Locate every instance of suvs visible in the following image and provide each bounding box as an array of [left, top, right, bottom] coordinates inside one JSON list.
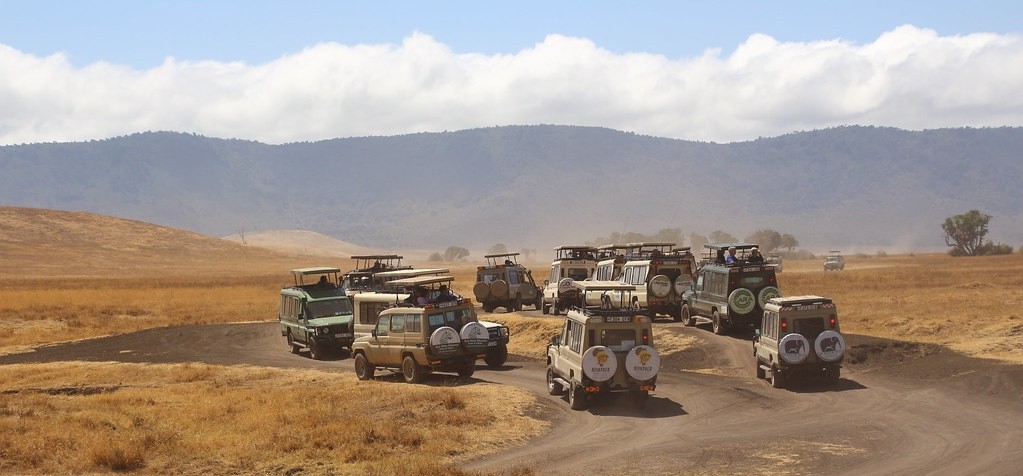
[[472, 252, 545, 313], [823, 250, 846, 271], [753, 295, 846, 387], [279, 267, 353, 359], [334, 252, 510, 370], [351, 275, 490, 384], [764, 249, 784, 274], [540, 240, 701, 321], [547, 281, 660, 411], [681, 242, 783, 334]]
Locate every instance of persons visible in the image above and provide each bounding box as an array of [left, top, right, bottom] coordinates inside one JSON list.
[[415, 287, 427, 304], [748, 247, 763, 264], [437, 285, 450, 300], [317, 276, 337, 296], [727, 247, 738, 263]]
